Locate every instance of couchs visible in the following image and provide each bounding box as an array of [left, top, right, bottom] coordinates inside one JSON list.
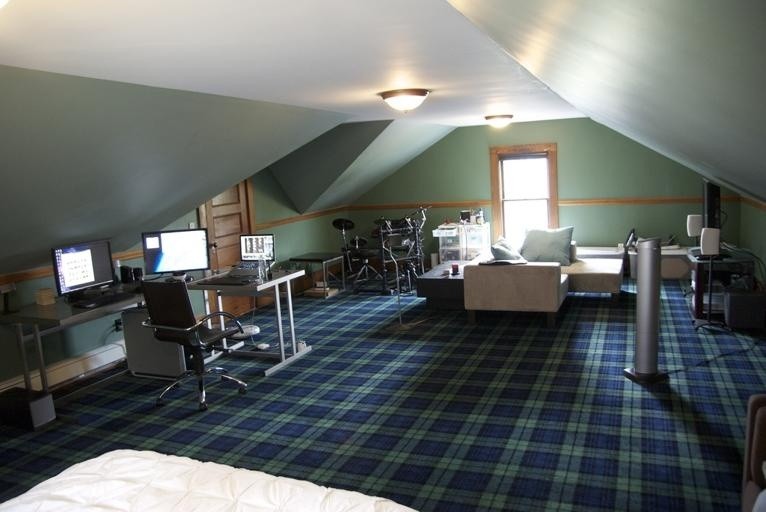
[[463, 241, 624, 322]]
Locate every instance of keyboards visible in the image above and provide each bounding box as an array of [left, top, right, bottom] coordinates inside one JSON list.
[[73, 293, 135, 309]]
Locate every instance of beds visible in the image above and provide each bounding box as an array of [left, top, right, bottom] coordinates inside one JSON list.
[[0, 449, 419, 512]]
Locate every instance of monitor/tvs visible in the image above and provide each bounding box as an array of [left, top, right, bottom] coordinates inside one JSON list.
[[692, 176, 732, 260], [142, 228, 210, 282], [50, 237, 115, 303]]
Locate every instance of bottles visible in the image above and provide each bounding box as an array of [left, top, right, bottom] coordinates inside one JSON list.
[[113, 259, 121, 281], [258, 254, 267, 280]]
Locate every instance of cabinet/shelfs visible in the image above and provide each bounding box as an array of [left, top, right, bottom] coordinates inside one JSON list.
[[465, 222, 490, 261], [432, 222, 465, 263]]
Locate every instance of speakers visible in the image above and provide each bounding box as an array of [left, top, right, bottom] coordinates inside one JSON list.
[[687, 214, 702, 237], [700, 228, 720, 255], [0, 387, 56, 431], [723, 290, 766, 329], [120, 265, 133, 283], [133, 267, 142, 281]]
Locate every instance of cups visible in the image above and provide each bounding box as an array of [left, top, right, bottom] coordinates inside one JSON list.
[[442, 269, 449, 275], [452, 264, 458, 273], [476, 215, 482, 225]]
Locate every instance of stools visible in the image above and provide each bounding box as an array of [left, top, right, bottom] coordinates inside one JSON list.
[[353, 249, 384, 285]]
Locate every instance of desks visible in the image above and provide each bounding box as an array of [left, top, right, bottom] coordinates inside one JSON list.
[[289, 252, 345, 300], [0, 269, 229, 392], [687, 246, 755, 319], [187, 269, 312, 376]]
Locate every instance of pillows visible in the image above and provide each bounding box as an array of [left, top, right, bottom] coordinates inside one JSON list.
[[491, 237, 525, 261], [521, 225, 574, 266]]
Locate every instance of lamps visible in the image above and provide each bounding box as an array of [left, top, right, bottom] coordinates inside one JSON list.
[[486, 115, 513, 129], [380, 89, 429, 110], [692, 228, 725, 331], [683, 214, 703, 297], [388, 234, 413, 331]]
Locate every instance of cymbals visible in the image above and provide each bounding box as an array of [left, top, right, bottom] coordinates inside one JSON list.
[[374, 219, 400, 226], [332, 219, 354, 230], [350, 238, 368, 246]]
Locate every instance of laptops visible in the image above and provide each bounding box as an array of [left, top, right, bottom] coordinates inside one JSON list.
[[228, 234, 275, 276]]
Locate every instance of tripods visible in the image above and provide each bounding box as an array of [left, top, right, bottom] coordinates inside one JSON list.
[[695, 253, 725, 331]]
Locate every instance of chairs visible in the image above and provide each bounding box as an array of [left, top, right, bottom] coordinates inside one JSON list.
[[141, 281, 248, 410]]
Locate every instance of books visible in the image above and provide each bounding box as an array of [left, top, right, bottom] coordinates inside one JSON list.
[[660, 241, 680, 251]]
